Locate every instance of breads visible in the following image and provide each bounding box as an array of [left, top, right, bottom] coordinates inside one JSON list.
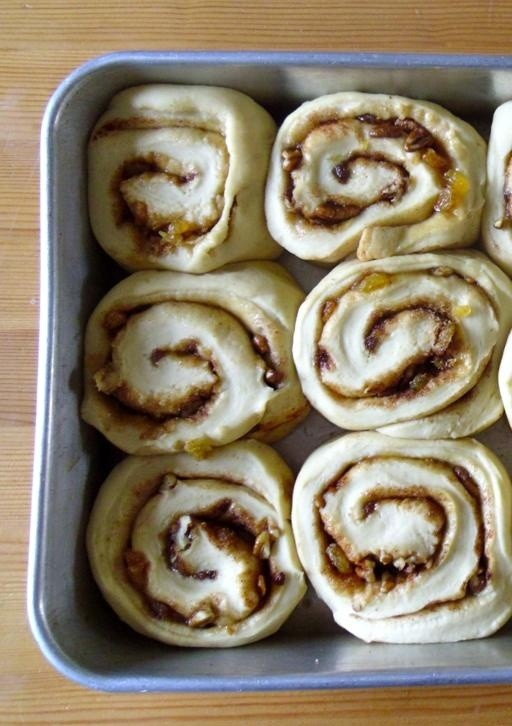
[[86, 439, 308, 648], [482, 100, 511, 273], [291, 432, 511, 643], [497, 327, 512, 432], [265, 90, 489, 263], [291, 249, 511, 440], [80, 258, 313, 457], [86, 84, 283, 275]]
